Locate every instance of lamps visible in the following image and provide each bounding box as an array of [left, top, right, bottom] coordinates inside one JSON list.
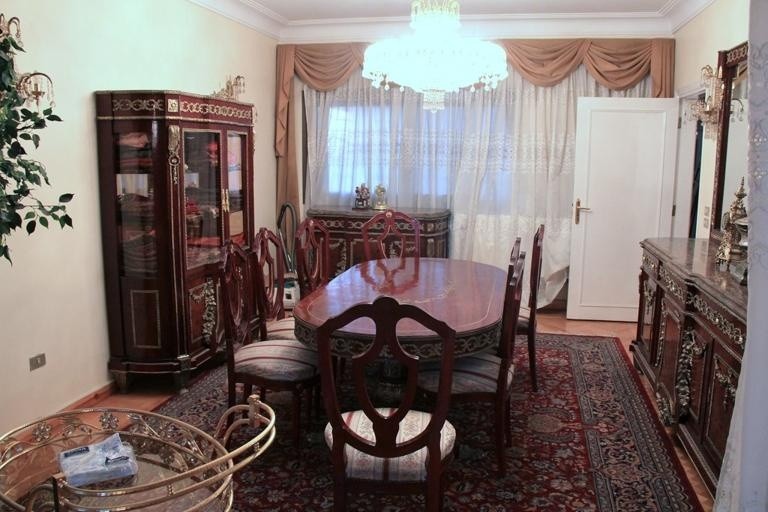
[[682, 65, 725, 142], [360, 0, 510, 115], [0, 10, 57, 112], [730, 97, 745, 123], [207, 74, 258, 152]]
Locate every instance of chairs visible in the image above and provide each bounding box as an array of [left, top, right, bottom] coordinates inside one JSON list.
[[313, 295, 461, 510], [359, 210, 422, 262], [483, 236, 523, 355], [251, 225, 296, 342], [417, 249, 528, 475], [294, 217, 333, 302], [514, 221, 545, 392], [217, 239, 321, 461]]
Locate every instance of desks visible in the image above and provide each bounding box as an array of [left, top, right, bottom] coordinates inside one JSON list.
[[290, 252, 508, 402], [628, 237, 749, 502]]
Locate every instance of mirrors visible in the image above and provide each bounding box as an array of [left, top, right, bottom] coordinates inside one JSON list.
[[710, 40, 748, 244]]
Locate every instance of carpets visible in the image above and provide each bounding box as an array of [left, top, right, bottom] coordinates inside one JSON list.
[[0, 332, 705, 512]]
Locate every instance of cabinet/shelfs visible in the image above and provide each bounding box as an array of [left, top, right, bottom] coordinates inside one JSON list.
[[305, 207, 452, 287], [95, 90, 260, 398]]
